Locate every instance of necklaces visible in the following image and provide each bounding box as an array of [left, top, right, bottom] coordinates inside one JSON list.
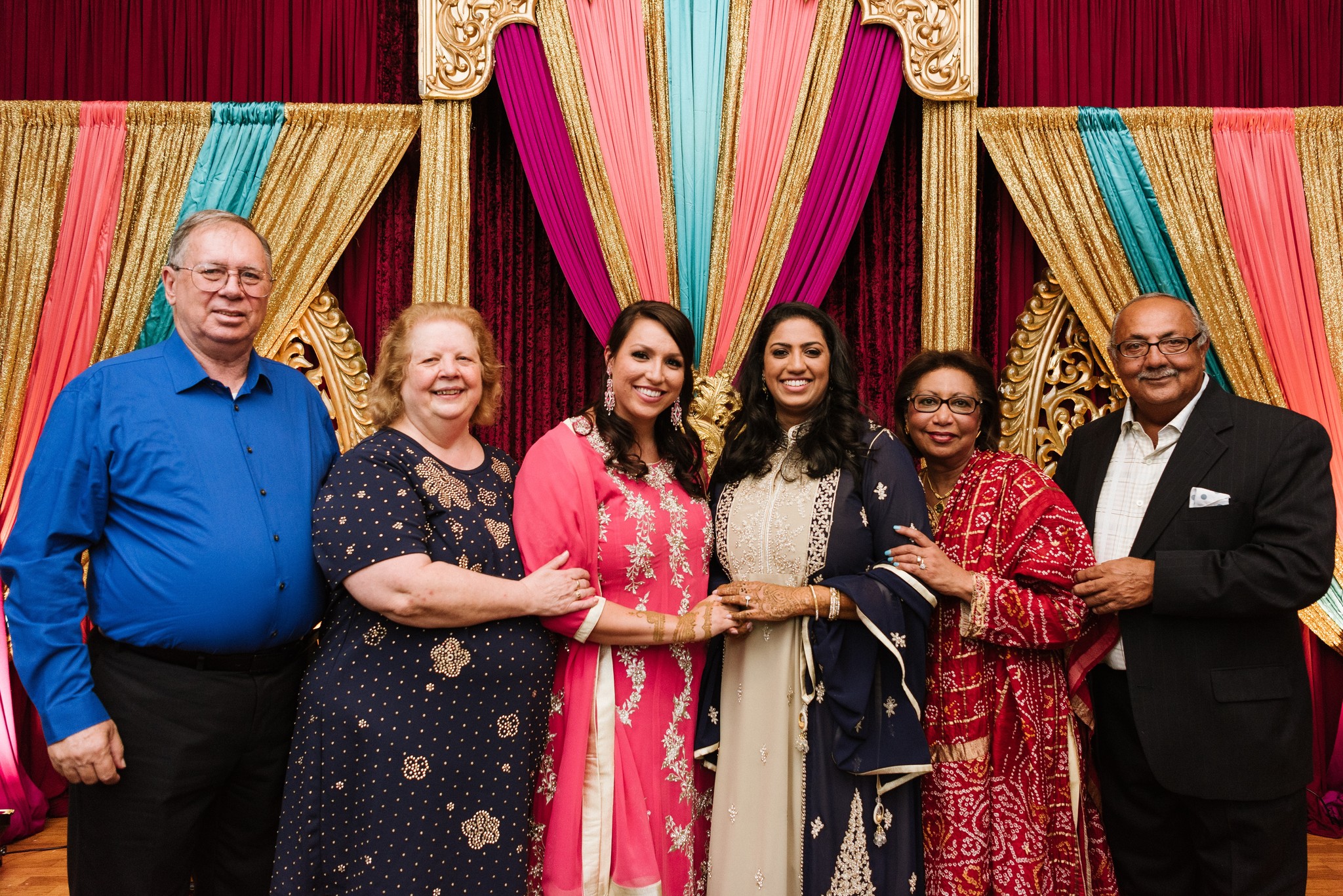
[[924, 467, 957, 517]]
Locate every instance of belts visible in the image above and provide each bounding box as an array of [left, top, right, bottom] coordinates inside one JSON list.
[[108, 637, 311, 676]]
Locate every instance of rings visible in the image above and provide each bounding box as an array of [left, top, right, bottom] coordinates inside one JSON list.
[[575, 590, 581, 601], [744, 595, 751, 606], [919, 559, 925, 569], [916, 555, 922, 565], [575, 579, 580, 591]]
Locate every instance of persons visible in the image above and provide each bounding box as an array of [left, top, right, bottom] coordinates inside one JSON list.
[[883, 349, 1122, 896], [1051, 292, 1337, 896], [700, 301, 938, 896], [0, 207, 343, 896], [512, 298, 745, 895], [269, 301, 599, 896]]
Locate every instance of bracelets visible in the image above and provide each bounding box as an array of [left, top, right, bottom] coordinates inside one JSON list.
[[808, 584, 818, 621], [827, 587, 840, 621]]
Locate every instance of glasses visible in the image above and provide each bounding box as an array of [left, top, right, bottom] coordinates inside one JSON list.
[[168, 263, 278, 298], [1110, 330, 1203, 358], [907, 394, 983, 415]]
